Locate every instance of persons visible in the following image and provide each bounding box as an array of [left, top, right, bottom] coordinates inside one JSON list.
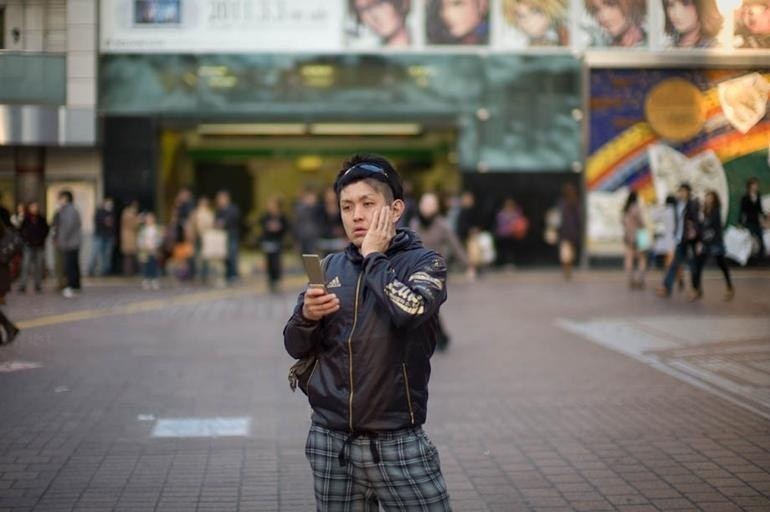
[[425, 2, 491, 44], [350, 2, 415, 48], [585, 0, 650, 49], [1, 190, 83, 344], [280, 155, 453, 512], [502, 1, 572, 47], [620, 176, 770, 302], [662, 0, 726, 48], [84, 184, 353, 293], [395, 178, 581, 350], [735, 0, 770, 48]]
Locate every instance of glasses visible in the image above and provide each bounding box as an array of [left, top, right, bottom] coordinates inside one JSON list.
[[337, 163, 389, 183]]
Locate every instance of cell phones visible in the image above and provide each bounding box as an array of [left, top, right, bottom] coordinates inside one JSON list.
[[302, 254, 330, 294]]
